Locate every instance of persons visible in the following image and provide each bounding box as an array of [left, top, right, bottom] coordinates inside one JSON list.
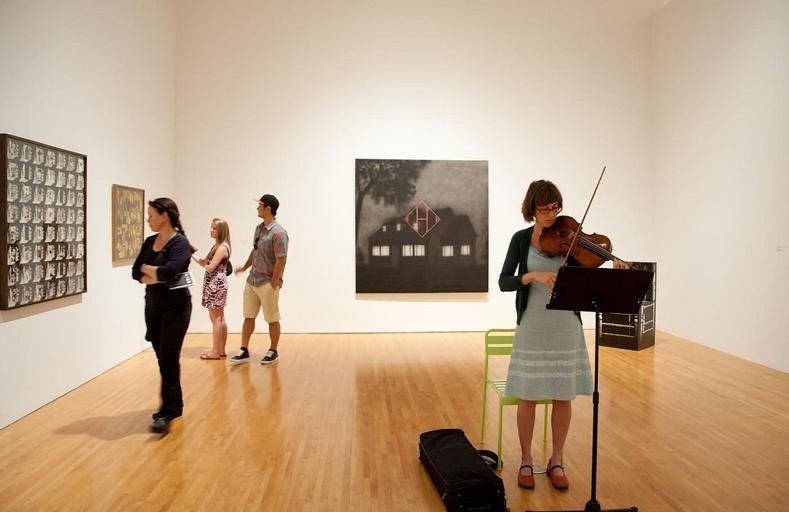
[[131, 197, 196, 435], [190, 217, 232, 360], [498, 181, 631, 491], [229, 194, 289, 364]]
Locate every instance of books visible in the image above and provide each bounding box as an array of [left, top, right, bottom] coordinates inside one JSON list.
[[167, 273, 195, 290]]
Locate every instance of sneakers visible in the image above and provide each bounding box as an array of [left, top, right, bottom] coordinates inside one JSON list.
[[260, 349, 279, 363], [229, 348, 250, 362]]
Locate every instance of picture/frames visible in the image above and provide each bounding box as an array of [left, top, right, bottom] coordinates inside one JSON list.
[[0, 132, 89, 311], [112, 183, 145, 262]]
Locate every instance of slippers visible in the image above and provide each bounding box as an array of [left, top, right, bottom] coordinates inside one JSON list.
[[200, 353, 226, 360]]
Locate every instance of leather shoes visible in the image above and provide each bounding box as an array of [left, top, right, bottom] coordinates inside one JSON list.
[[152, 406, 182, 419], [547, 458, 569, 490], [149, 417, 169, 432], [518, 464, 535, 488]]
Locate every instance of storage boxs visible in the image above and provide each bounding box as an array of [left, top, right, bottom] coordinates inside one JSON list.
[[598, 261, 658, 351]]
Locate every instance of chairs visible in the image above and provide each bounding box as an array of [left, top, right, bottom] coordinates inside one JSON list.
[[480, 327, 565, 472]]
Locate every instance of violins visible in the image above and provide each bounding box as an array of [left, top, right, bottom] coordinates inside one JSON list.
[[539, 216, 639, 271]]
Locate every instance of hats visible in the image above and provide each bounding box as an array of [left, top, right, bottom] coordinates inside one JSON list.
[[252, 194, 279, 210]]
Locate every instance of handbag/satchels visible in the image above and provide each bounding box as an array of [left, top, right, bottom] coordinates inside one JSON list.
[[419, 428, 506, 511], [226, 261, 233, 276]]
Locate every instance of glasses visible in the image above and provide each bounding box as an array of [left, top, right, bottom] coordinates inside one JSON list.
[[253, 236, 260, 251], [535, 205, 562, 214]]
